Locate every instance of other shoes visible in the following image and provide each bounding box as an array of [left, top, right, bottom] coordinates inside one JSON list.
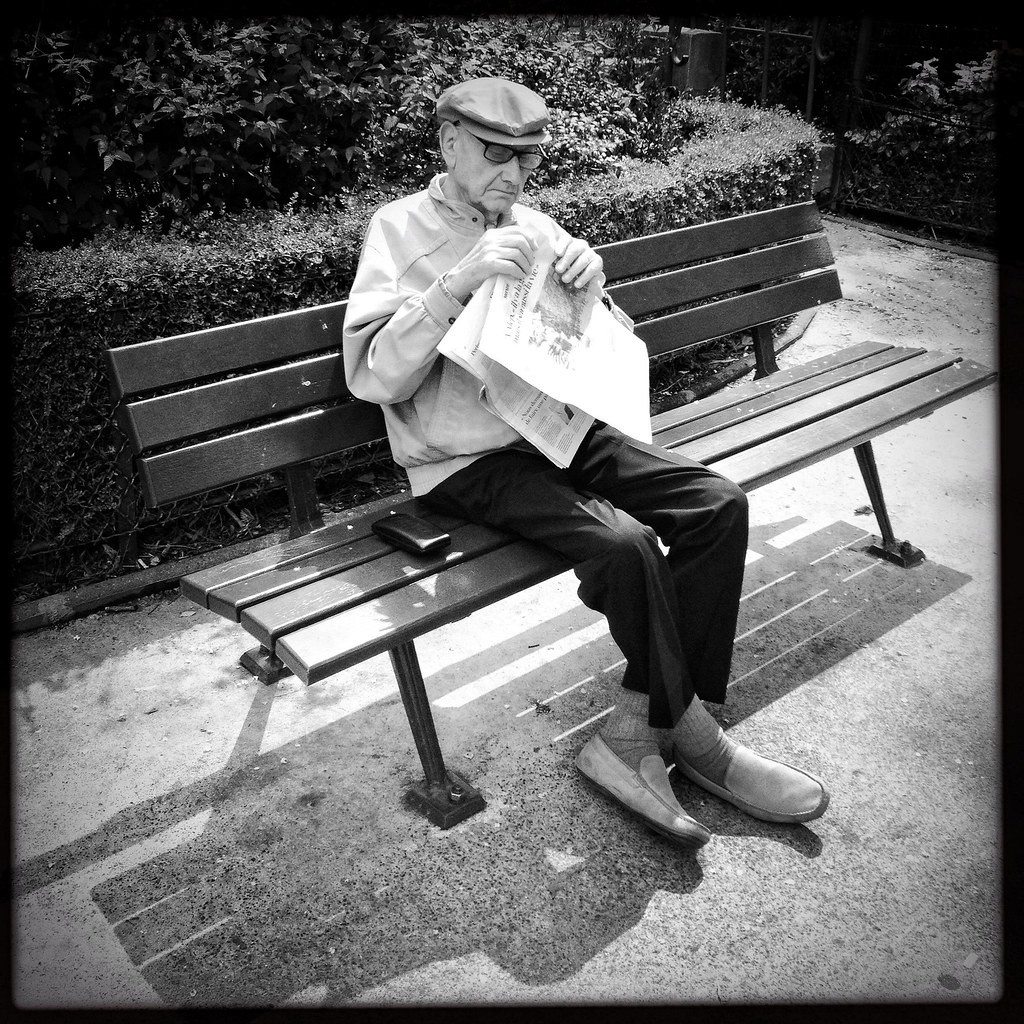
[[673, 731, 829, 823], [573, 732, 711, 849]]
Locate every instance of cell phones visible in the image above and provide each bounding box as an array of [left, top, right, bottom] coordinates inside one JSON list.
[[371, 513, 451, 555]]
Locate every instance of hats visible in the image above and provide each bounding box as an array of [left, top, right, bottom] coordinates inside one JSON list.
[[436, 78, 554, 145]]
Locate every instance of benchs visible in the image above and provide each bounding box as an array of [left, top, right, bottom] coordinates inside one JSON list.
[[105, 199, 998, 830]]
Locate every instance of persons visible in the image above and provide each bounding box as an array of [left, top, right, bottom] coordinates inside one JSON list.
[[343, 78, 830, 845]]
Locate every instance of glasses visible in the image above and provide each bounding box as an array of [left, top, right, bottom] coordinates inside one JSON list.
[[469, 131, 549, 171]]
